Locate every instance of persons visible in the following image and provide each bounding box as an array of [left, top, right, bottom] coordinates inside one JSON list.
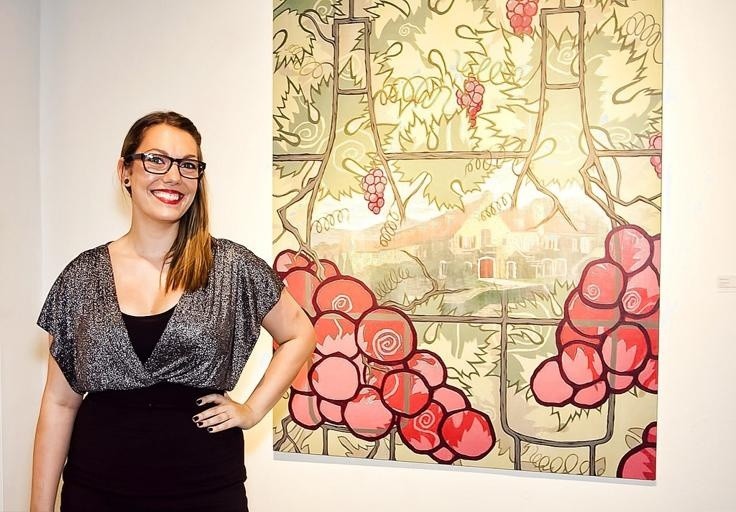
[[30, 112, 317, 512]]
